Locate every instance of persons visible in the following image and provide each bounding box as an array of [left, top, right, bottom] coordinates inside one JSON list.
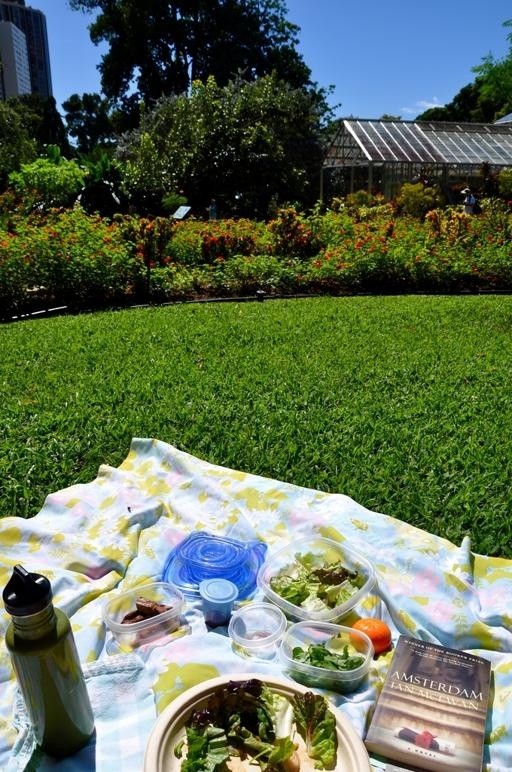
[[461, 187, 475, 215], [208, 199, 218, 220]]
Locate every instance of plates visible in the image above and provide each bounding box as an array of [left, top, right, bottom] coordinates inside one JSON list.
[[144, 673, 375, 772]]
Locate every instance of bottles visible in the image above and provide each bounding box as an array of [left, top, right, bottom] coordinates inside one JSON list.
[[1, 563, 100, 758]]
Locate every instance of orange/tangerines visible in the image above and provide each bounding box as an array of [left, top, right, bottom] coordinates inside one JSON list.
[[350, 617, 391, 654]]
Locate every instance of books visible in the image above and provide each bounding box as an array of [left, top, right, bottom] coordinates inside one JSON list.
[[364, 636, 491, 772]]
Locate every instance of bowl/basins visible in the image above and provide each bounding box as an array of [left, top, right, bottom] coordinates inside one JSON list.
[[104, 581, 187, 648], [257, 535, 377, 625], [227, 602, 286, 663], [280, 620, 375, 693]]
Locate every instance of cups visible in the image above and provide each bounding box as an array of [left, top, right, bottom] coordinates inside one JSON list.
[[200, 577, 238, 627]]
